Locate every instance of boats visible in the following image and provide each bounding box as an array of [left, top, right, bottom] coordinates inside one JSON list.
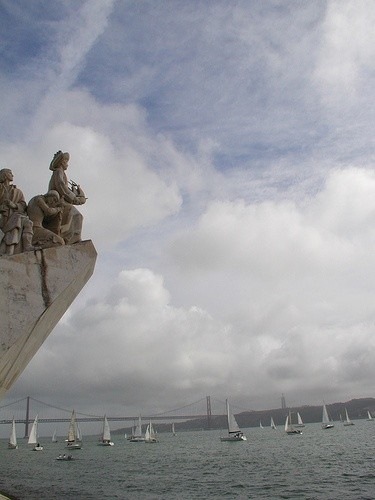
[[57, 453, 73, 459]]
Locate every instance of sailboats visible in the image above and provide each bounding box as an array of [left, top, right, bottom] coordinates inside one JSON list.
[[285, 416, 301, 435], [344, 407, 354, 426], [125, 414, 162, 444], [367, 411, 373, 420], [98, 414, 115, 446], [320, 399, 334, 429], [294, 412, 305, 428], [65, 408, 82, 450], [9, 415, 19, 449], [259, 420, 264, 429], [27, 414, 45, 451], [51, 429, 57, 443], [270, 416, 277, 431], [218, 398, 245, 442], [338, 413, 343, 422], [172, 423, 176, 437]]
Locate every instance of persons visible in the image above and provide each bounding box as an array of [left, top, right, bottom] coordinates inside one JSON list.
[[0, 167, 41, 256], [26, 190, 66, 250], [48, 150, 86, 244]]
